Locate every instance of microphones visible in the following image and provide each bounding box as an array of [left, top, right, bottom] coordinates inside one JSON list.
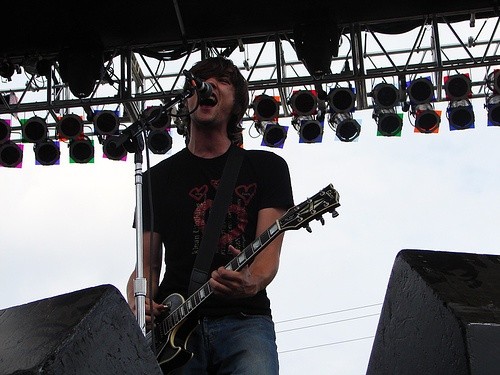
[[182, 70, 213, 98]]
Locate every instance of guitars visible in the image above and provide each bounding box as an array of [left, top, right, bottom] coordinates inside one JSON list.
[[143, 182, 341, 375]]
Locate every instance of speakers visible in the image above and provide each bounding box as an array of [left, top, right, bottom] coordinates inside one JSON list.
[[365, 248, 500, 375], [0, 284, 163, 375]]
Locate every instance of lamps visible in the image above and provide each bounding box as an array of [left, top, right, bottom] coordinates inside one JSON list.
[[57, 34, 104, 98], [0, 69, 500, 168], [295, 22, 340, 79]]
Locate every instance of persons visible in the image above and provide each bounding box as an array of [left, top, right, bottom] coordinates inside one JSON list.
[[127, 57, 294, 375]]
